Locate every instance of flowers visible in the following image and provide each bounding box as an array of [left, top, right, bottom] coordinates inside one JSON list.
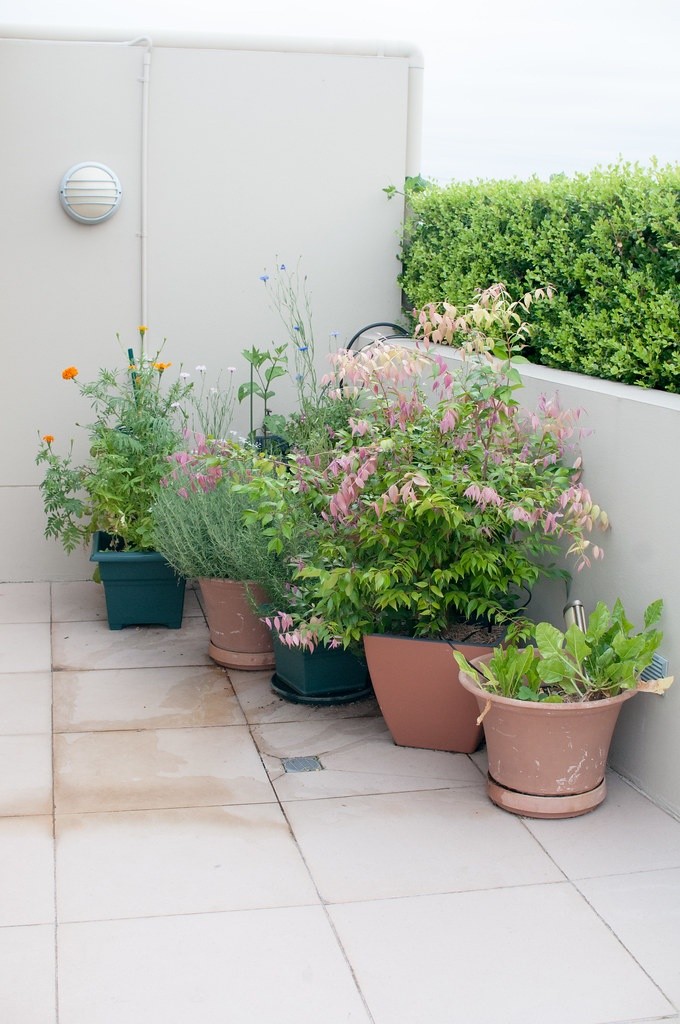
[[35, 255, 614, 655]]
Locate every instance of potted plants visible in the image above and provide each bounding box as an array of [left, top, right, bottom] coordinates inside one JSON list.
[[455, 598, 671, 821]]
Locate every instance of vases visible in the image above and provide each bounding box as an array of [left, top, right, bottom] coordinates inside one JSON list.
[[89, 527, 187, 631], [358, 614, 525, 753], [192, 572, 284, 669], [266, 613, 374, 704]]
